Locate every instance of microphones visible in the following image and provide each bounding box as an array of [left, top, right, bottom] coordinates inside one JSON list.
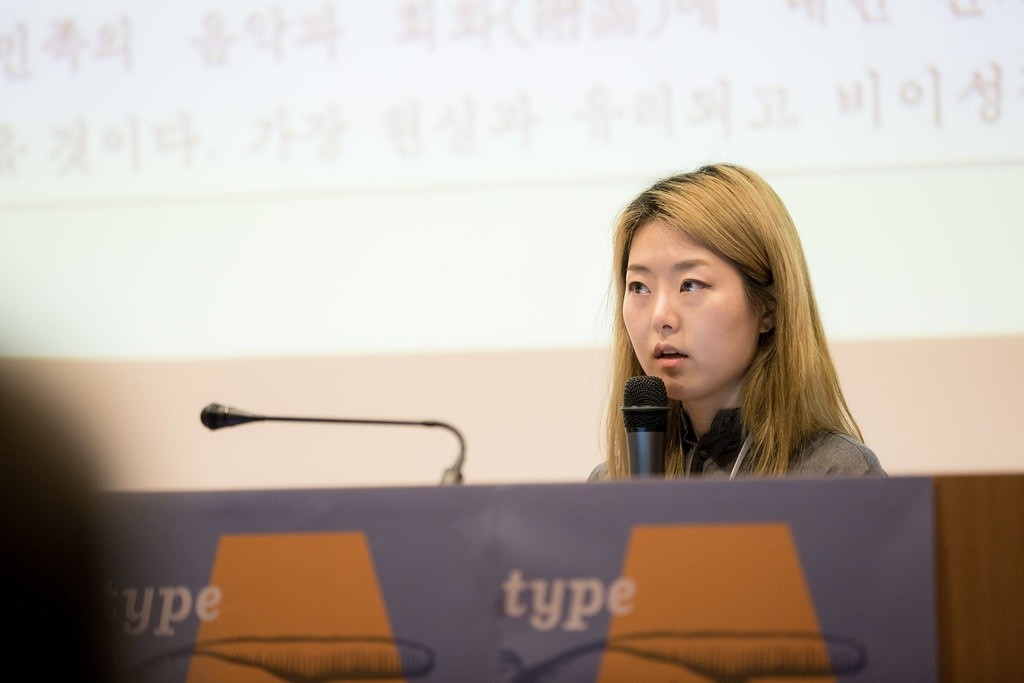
[[621, 375, 672, 481], [200, 402, 466, 485]]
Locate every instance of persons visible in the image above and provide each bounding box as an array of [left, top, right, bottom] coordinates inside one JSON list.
[[584, 164, 889, 481], [0, 349, 147, 683]]
